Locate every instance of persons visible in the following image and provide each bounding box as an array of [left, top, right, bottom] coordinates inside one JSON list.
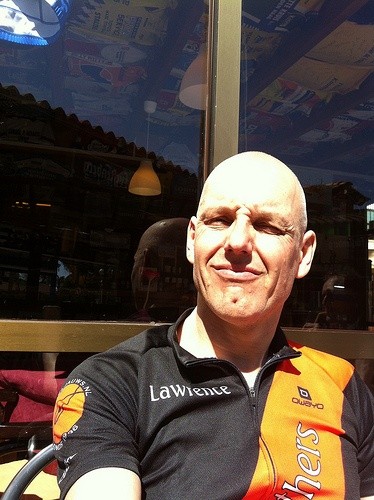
[[302, 269, 367, 331], [124, 217, 196, 323], [53, 152, 374, 500]]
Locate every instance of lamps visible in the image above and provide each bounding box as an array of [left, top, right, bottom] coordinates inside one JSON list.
[[128, 101, 161, 196]]
